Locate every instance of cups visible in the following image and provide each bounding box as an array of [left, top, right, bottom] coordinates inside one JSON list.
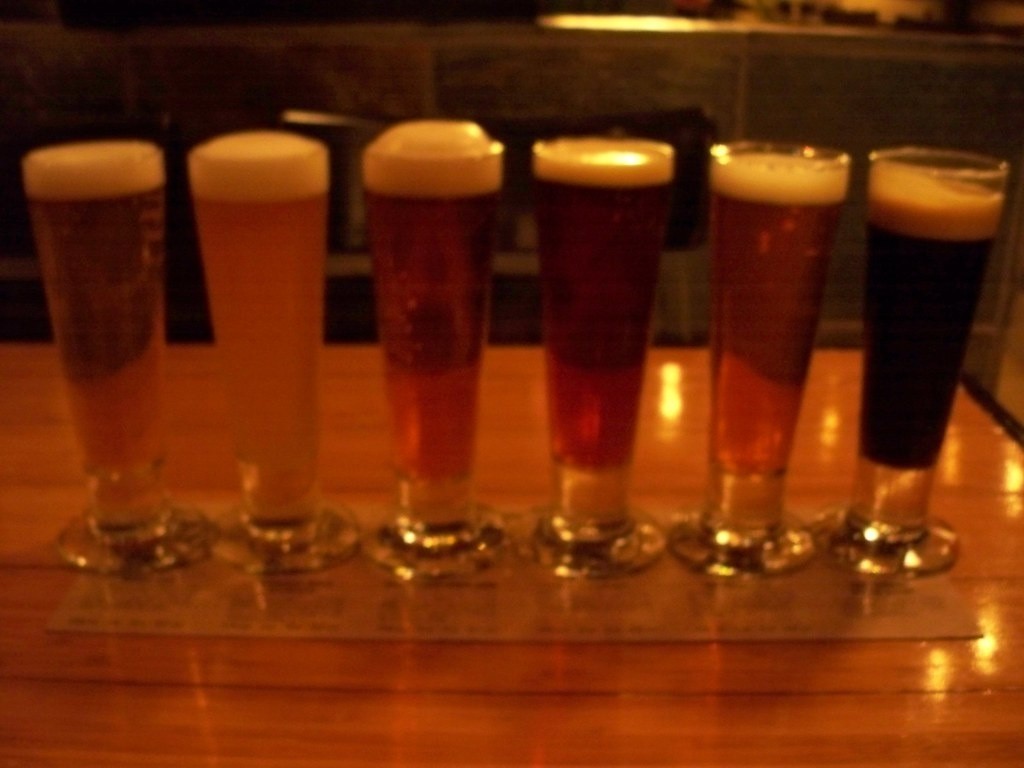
[[518, 138, 676, 580], [190, 135, 359, 575], [358, 144, 508, 586], [23, 139, 216, 576], [804, 147, 1009, 574], [666, 138, 852, 583]]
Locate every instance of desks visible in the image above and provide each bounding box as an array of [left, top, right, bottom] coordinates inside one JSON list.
[[0, 277, 1024, 768]]
